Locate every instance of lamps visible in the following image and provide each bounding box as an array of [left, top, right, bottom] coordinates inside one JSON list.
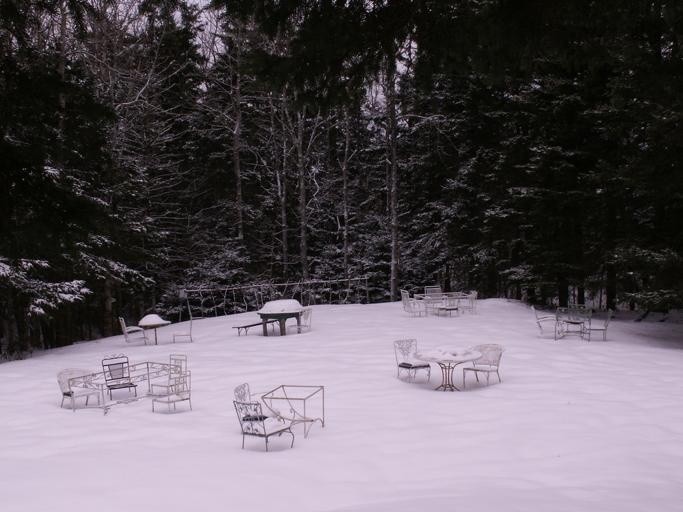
[[173, 316, 193, 343], [119, 317, 149, 345], [393, 339, 430, 383], [463, 344, 502, 388], [57, 354, 192, 415], [287, 310, 311, 334], [234, 383, 273, 434], [233, 401, 294, 452], [400, 285, 478, 318], [531, 304, 613, 341]]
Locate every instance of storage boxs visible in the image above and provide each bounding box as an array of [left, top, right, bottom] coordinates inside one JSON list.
[[232, 320, 279, 336]]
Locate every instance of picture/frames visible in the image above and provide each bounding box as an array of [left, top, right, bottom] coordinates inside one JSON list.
[[257, 311, 305, 337], [413, 346, 482, 392], [138, 323, 169, 345]]
